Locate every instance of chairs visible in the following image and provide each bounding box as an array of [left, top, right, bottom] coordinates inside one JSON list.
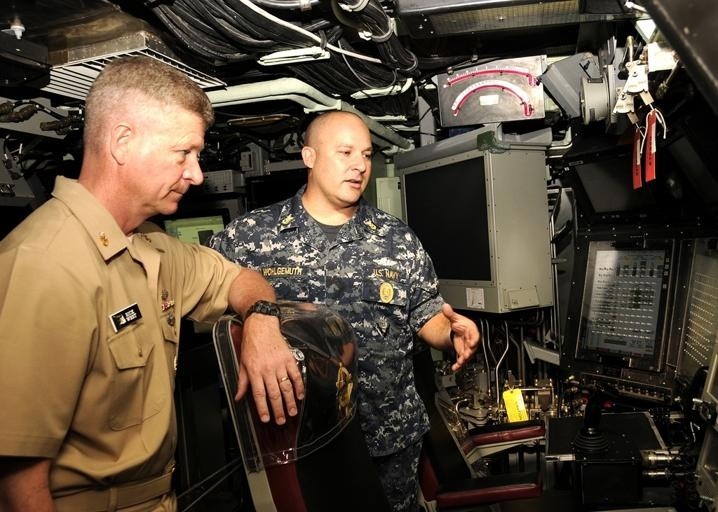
[[212, 301, 394, 512], [413, 335, 545, 511]]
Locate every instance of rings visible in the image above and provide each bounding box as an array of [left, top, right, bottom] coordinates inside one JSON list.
[[278, 376, 289, 384]]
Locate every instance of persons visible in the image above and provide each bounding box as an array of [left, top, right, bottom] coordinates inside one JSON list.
[[0, 50, 306, 511], [205, 111, 480, 511]]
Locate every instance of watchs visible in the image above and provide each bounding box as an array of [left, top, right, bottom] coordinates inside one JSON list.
[[243, 300, 282, 329]]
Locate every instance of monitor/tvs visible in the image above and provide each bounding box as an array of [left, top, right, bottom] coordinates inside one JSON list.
[[157, 209, 232, 249], [402, 154, 494, 287]]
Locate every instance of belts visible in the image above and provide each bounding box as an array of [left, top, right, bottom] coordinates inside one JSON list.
[[50, 461, 176, 512]]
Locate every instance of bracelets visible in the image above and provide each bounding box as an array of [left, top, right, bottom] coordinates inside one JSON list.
[[450, 330, 455, 343]]
